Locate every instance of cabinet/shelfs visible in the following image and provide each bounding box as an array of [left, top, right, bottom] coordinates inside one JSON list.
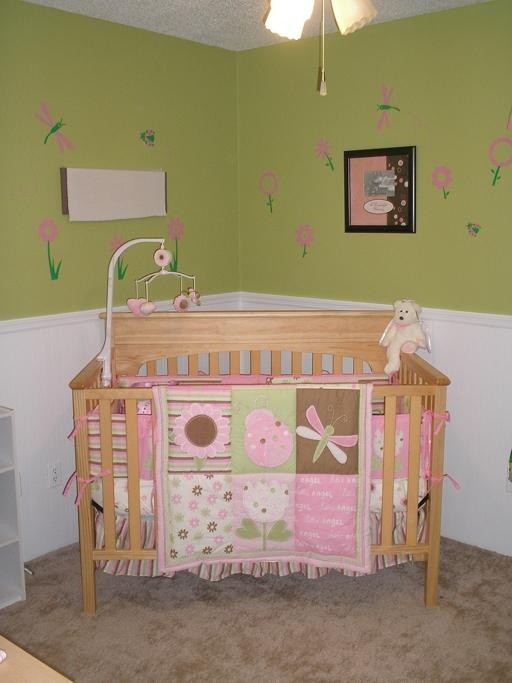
[[0, 407, 28, 613]]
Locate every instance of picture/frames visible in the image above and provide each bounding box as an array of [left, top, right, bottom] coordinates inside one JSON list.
[[343, 145, 418, 234]]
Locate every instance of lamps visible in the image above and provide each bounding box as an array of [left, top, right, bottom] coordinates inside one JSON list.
[[262, 0, 379, 97]]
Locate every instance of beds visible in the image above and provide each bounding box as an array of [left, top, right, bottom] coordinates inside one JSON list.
[[67, 306, 453, 615]]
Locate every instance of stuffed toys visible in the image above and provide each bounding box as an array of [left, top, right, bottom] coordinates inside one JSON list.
[[380, 297, 431, 375]]
